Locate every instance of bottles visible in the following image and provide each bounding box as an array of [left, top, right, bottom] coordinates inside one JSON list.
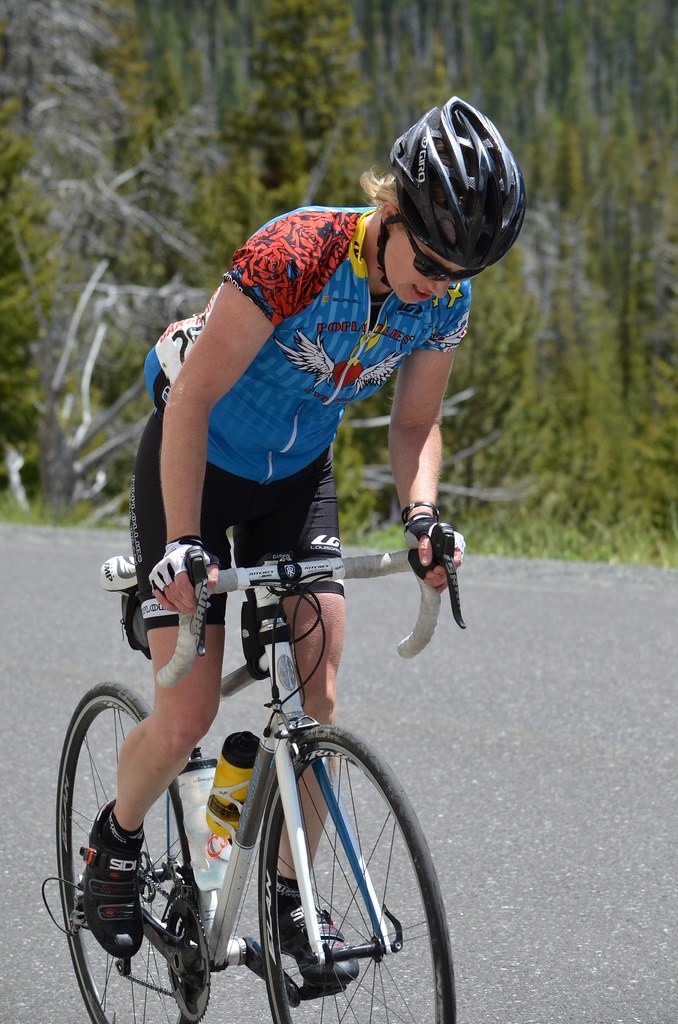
[[178, 747, 233, 892], [206, 731, 260, 838]]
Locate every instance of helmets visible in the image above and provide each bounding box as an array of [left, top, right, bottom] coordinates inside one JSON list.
[[389, 95, 527, 271]]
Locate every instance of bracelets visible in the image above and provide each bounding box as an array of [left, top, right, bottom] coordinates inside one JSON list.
[[402, 501, 439, 524]]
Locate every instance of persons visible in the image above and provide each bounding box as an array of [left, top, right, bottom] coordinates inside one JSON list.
[[82, 97, 522, 984]]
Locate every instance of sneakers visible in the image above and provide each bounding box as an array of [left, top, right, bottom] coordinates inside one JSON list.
[[278, 892, 360, 987], [79, 799, 145, 959]]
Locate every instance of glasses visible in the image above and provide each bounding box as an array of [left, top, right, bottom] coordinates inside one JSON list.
[[398, 214, 486, 284]]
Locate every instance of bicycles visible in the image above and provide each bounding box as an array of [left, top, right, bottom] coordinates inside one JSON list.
[[40, 520, 467, 1024]]
